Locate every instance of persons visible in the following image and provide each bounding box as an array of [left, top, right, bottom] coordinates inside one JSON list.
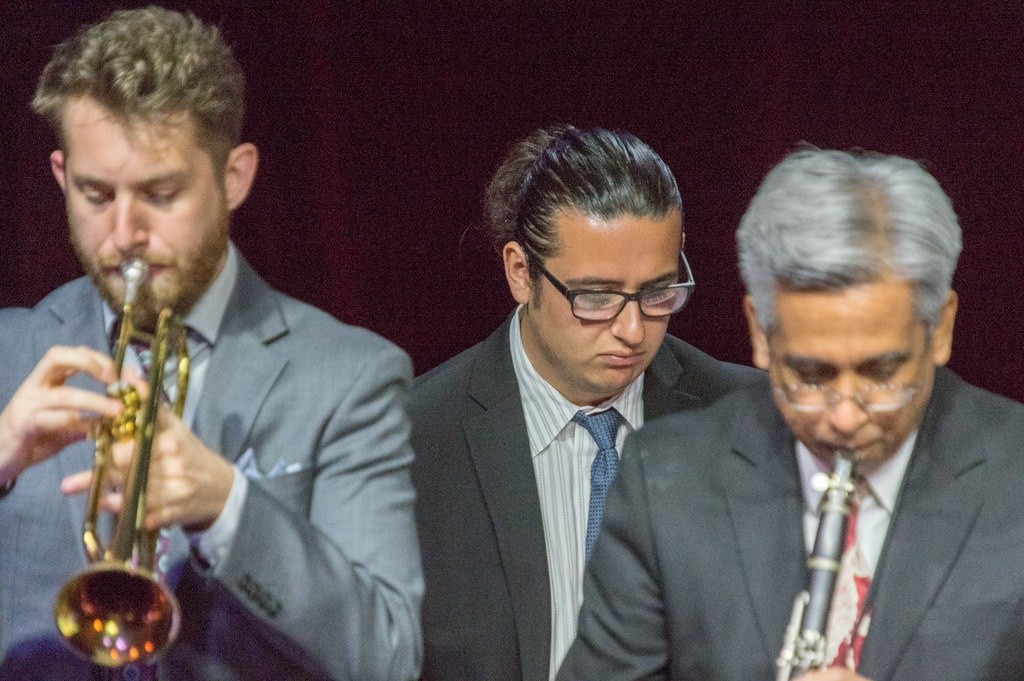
[[0, 2, 424, 681], [409, 121, 783, 679], [550, 141, 1023, 679]]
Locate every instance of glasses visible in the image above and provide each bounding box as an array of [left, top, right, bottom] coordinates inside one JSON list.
[[762, 333, 927, 415], [526, 245, 699, 322]]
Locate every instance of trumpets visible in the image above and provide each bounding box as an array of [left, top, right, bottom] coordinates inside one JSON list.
[[56, 259, 188, 668]]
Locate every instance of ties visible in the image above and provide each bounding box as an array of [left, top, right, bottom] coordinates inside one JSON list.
[[823, 476, 874, 674], [109, 339, 170, 680], [570, 408, 622, 565]]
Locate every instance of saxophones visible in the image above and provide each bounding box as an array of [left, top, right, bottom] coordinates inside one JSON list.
[[789, 446, 856, 681]]
